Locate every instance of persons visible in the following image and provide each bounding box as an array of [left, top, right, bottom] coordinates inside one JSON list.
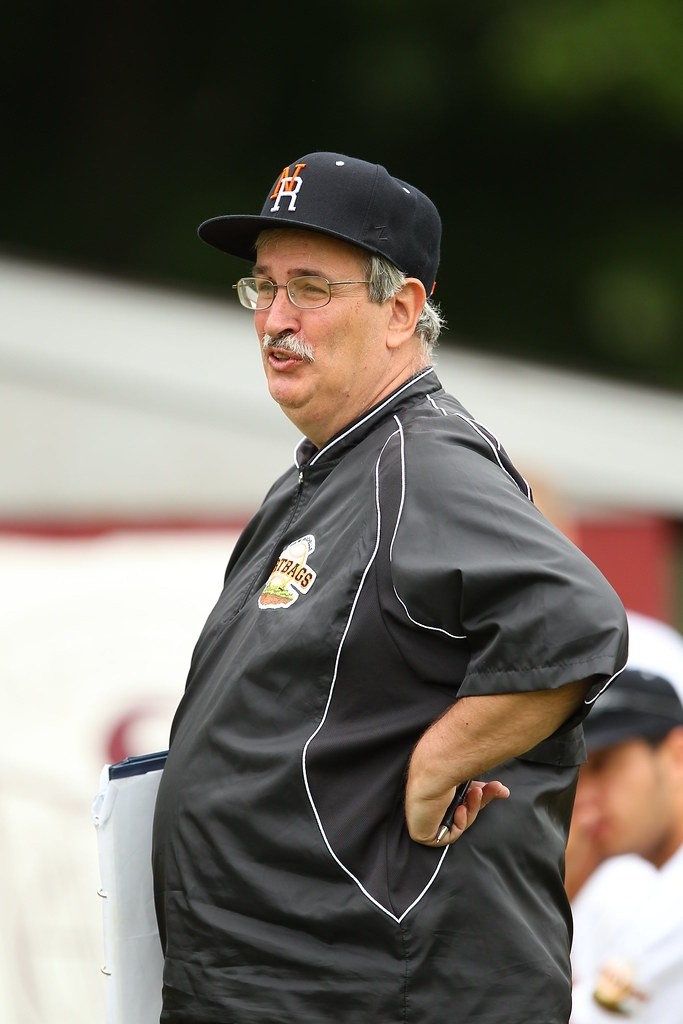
[[563, 668, 683, 1024], [151, 150, 628, 1024]]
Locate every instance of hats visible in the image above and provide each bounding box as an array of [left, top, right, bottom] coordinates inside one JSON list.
[[581, 669, 682, 752], [198, 152, 441, 296]]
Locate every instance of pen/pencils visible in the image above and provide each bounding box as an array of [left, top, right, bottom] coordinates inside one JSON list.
[[436, 776, 472, 845]]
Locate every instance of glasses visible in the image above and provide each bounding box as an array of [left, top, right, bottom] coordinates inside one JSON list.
[[232, 275, 370, 311]]
